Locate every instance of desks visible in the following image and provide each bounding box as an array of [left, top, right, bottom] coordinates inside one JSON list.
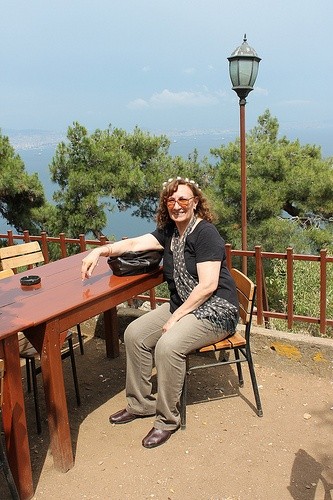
[[0, 249, 165, 500]]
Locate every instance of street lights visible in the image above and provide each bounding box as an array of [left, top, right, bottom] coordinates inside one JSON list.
[[226, 32, 263, 276]]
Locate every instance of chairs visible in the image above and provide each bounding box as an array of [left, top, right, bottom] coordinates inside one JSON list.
[[182, 268, 263, 430], [0, 242, 80, 434]]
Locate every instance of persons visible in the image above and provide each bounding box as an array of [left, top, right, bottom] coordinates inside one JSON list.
[[80, 177, 239, 448]]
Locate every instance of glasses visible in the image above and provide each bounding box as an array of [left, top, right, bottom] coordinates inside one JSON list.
[[166, 196, 195, 208]]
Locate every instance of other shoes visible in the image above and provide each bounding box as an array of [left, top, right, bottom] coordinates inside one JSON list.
[[142, 423, 180, 448]]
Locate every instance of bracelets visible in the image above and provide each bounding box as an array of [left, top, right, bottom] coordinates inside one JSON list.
[[102, 244, 112, 257]]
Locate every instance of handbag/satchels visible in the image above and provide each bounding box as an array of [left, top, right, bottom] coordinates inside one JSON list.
[[107, 250, 164, 276]]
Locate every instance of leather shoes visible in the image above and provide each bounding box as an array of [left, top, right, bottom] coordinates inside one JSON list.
[[109, 408, 156, 424]]
